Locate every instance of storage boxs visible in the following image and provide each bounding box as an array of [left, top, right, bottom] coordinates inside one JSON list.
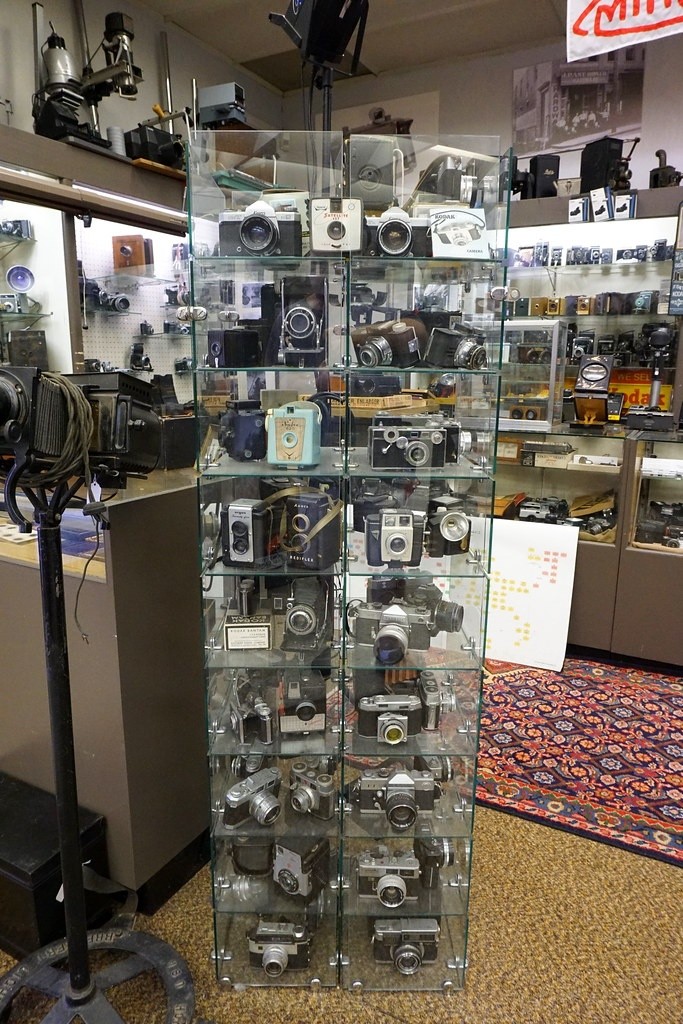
[[0, 773, 112, 964]]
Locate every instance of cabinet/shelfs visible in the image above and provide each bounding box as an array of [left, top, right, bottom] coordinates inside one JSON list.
[[179, 124, 519, 995], [501, 256, 678, 370], [492, 427, 641, 654], [612, 431, 683, 665], [0, 469, 202, 897]]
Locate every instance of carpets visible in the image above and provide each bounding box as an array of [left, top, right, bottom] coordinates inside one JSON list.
[[472, 657, 683, 869]]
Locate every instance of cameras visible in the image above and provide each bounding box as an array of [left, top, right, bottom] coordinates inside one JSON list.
[[78, 235, 195, 375], [0, 220, 31, 240], [509, 238, 676, 430], [0, 294, 20, 313], [208, 134, 491, 978]]
